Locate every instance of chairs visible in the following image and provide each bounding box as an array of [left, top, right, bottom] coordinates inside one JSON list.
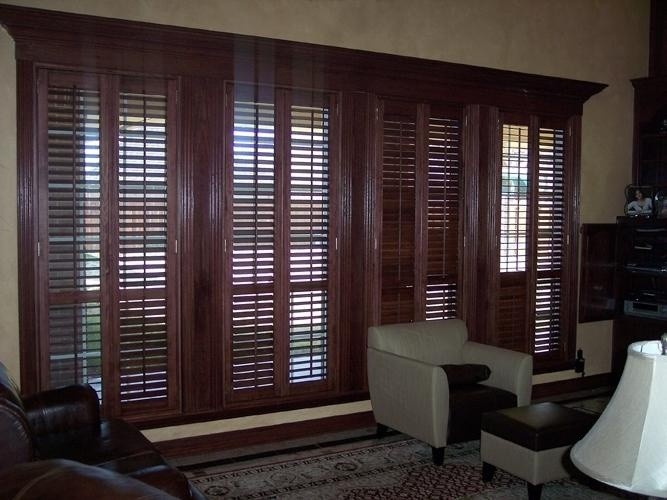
[[367, 320, 534, 466]]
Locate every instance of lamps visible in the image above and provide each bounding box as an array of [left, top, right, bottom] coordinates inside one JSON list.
[[570, 333, 667, 500]]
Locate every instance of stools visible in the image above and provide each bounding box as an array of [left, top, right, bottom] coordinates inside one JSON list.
[[480, 402, 597, 500]]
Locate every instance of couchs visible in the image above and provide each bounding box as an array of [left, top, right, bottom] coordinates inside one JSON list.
[[0, 359, 194, 500]]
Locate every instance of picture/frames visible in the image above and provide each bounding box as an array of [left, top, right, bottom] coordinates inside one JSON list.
[[624, 184, 655, 217]]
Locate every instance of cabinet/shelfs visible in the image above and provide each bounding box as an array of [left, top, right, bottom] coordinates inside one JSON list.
[[580, 216, 667, 373]]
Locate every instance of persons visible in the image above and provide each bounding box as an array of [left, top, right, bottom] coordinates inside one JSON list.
[[625, 188, 652, 215]]
